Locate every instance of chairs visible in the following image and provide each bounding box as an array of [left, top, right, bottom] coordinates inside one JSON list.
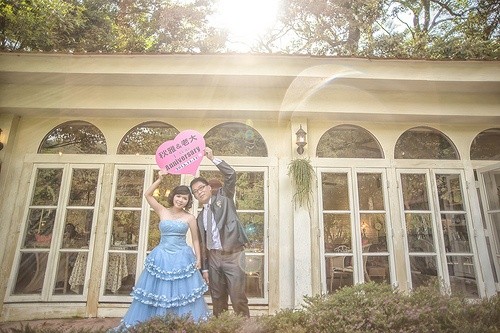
[[329, 243, 372, 292]]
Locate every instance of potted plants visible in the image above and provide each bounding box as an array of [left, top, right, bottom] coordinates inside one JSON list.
[[286, 154, 315, 210]]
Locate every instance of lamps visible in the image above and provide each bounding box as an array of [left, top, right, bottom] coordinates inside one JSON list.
[[296, 124, 307, 155]]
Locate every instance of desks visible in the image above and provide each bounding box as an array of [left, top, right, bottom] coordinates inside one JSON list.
[[68, 244, 138, 295], [245, 253, 264, 297]]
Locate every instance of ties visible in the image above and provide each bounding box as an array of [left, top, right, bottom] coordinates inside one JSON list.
[[206, 204, 213, 251]]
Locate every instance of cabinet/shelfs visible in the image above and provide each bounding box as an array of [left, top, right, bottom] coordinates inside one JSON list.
[[439, 173, 476, 277]]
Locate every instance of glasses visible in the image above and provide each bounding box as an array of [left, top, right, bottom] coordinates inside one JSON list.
[[191, 185, 206, 195]]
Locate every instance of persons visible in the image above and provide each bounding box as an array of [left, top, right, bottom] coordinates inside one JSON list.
[[190, 146, 250, 319], [123, 168, 211, 326], [63, 223, 82, 241]]
